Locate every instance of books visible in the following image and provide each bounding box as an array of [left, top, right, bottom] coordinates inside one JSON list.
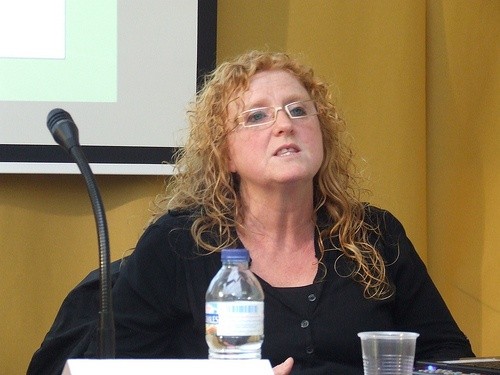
[[415, 356, 500, 375]]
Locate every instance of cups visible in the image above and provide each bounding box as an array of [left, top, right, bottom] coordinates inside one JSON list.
[[357, 331, 420, 375]]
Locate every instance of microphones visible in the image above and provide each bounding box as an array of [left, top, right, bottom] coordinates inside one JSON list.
[[46, 108, 117, 359]]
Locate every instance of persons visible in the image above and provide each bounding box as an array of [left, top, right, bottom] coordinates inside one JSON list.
[[83, 50, 476, 375]]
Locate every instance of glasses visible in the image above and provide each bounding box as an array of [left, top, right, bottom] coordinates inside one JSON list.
[[224, 98, 320, 138]]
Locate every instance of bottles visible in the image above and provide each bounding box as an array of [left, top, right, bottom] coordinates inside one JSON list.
[[205, 249, 265, 359]]
